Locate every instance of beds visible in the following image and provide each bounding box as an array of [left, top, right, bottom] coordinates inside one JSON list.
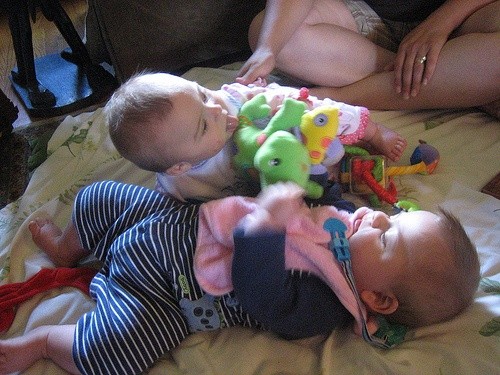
[[0, 67, 500, 375]]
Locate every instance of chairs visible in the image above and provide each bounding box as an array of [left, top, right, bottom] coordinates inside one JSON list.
[[92, 0, 259, 78]]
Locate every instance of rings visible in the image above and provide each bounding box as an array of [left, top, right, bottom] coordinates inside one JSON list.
[[414, 55, 427, 64]]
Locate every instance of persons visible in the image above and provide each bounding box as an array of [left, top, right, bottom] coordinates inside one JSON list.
[[233, 0, 500, 119], [106, 70, 408, 208], [0, 179, 482, 375]]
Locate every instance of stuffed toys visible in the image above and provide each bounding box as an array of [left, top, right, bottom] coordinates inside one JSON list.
[[232, 92, 342, 200]]
